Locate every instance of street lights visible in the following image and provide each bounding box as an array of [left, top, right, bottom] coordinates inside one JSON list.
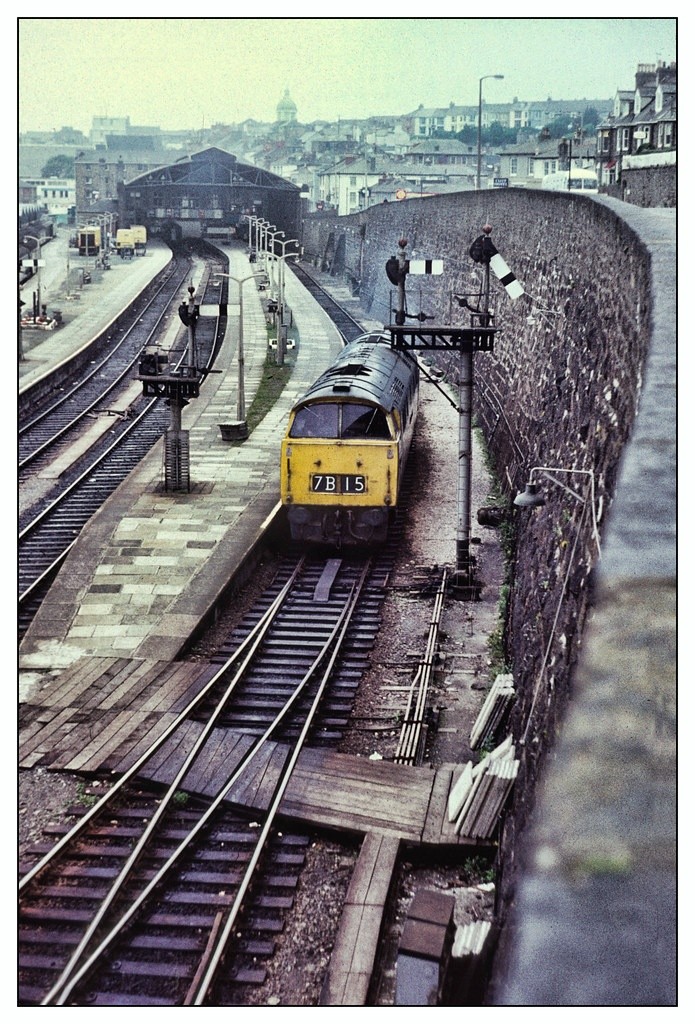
[[260, 230, 286, 299], [475, 74, 505, 190], [254, 251, 300, 367], [241, 214, 258, 252], [77, 211, 119, 276], [247, 216, 266, 264], [255, 224, 276, 282], [268, 238, 299, 351], [23, 235, 54, 317], [213, 271, 269, 421]]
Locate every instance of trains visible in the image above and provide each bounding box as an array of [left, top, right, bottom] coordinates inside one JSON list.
[[279, 328, 421, 553]]
